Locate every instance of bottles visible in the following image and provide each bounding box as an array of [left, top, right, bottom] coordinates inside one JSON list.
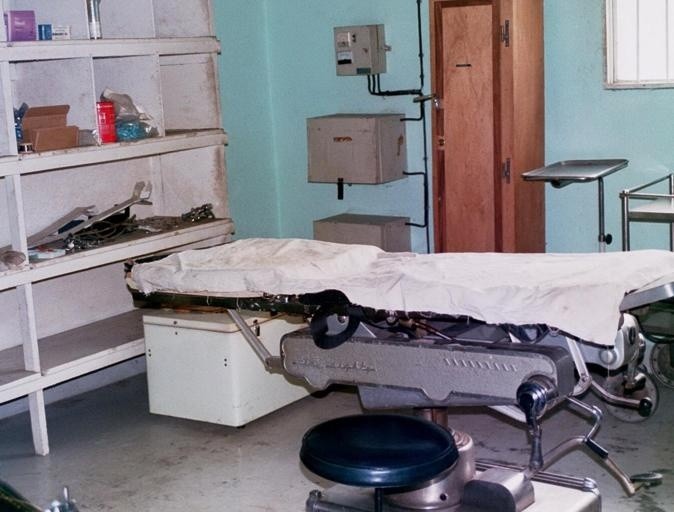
[[96, 102, 117, 143], [86, 0, 103, 39], [38, 24, 53, 41]]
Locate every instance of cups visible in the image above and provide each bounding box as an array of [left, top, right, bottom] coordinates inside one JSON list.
[[18, 143, 33, 154]]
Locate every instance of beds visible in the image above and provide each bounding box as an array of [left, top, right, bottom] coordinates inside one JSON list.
[[126, 235, 674, 497]]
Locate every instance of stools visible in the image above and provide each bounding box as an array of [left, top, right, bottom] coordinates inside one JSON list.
[[295, 411, 459, 512]]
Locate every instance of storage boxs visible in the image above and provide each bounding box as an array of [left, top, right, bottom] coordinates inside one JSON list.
[[141, 311, 312, 426]]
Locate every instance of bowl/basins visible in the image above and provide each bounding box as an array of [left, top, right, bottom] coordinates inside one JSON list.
[[115, 120, 145, 141]]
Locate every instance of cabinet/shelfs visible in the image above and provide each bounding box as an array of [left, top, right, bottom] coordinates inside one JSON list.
[[620, 172, 674, 251], [0, 0, 236, 455]]
